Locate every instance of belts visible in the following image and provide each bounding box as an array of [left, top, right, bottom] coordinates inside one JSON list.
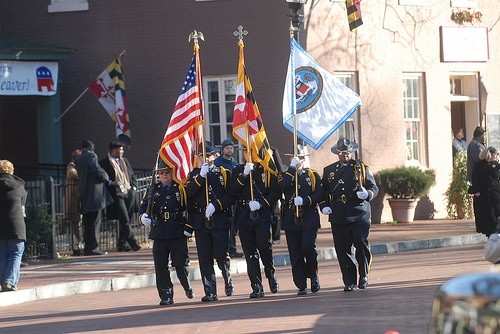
[[191, 206, 231, 215], [237, 199, 248, 207], [157, 211, 183, 220], [331, 194, 357, 204]]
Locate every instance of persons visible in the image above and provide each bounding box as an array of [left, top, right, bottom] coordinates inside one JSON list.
[[452, 127, 467, 166], [467, 127, 486, 194], [139, 161, 194, 305], [99, 140, 141, 252], [0, 159, 27, 290], [269, 146, 284, 243], [278, 144, 325, 295], [473, 147, 500, 239], [214, 138, 244, 258], [65, 150, 84, 256], [225, 145, 281, 298], [319, 138, 380, 291], [76, 140, 109, 256]]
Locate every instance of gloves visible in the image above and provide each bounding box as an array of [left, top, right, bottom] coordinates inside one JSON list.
[[322, 207, 332, 215], [294, 196, 303, 205], [141, 213, 152, 225], [291, 157, 300, 167], [205, 203, 215, 217], [184, 231, 191, 237], [244, 162, 254, 175], [356, 186, 369, 199], [249, 201, 260, 211], [200, 163, 209, 177]]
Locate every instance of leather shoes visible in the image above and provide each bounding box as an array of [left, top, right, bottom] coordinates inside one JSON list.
[[186, 289, 192, 299], [84, 247, 108, 255], [202, 294, 218, 302], [250, 292, 264, 298], [359, 277, 368, 289], [311, 277, 320, 292], [298, 288, 306, 295], [132, 244, 141, 251], [269, 278, 277, 293], [160, 298, 173, 305], [117, 244, 132, 252], [225, 286, 232, 296], [344, 284, 355, 291]]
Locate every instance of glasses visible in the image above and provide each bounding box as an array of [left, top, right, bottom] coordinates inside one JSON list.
[[158, 169, 171, 175]]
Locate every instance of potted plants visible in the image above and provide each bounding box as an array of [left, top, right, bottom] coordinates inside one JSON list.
[[444, 150, 475, 219], [377, 166, 435, 223]]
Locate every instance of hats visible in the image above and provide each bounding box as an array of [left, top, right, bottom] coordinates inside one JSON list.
[[158, 160, 169, 170], [222, 139, 234, 147], [242, 145, 252, 150], [109, 141, 125, 150], [198, 141, 217, 156], [285, 144, 310, 156], [331, 138, 358, 155], [473, 127, 486, 137]]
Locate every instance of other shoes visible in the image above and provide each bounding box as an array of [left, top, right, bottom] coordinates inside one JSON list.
[[74, 250, 85, 256], [230, 251, 243, 258], [185, 258, 189, 266], [272, 240, 279, 244], [2, 283, 16, 291]]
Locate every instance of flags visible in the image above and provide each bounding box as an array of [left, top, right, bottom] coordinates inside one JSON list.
[[284, 38, 362, 150], [232, 39, 279, 177], [160, 43, 206, 209], [186, 141, 232, 302], [345, 0, 363, 31], [89, 55, 132, 149]]
